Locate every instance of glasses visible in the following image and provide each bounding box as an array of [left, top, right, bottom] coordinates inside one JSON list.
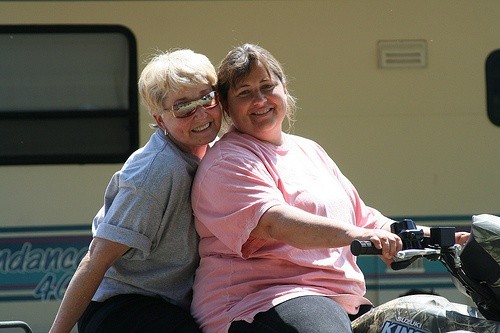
[[164, 91, 219, 118]]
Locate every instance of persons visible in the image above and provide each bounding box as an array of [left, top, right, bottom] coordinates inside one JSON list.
[[192, 42, 470, 333], [48, 48, 222, 333]]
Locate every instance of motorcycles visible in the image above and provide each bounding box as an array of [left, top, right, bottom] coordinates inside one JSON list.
[[349, 214, 500, 333]]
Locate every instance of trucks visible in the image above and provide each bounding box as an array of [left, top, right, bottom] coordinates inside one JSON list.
[[0, 0, 500, 332]]
